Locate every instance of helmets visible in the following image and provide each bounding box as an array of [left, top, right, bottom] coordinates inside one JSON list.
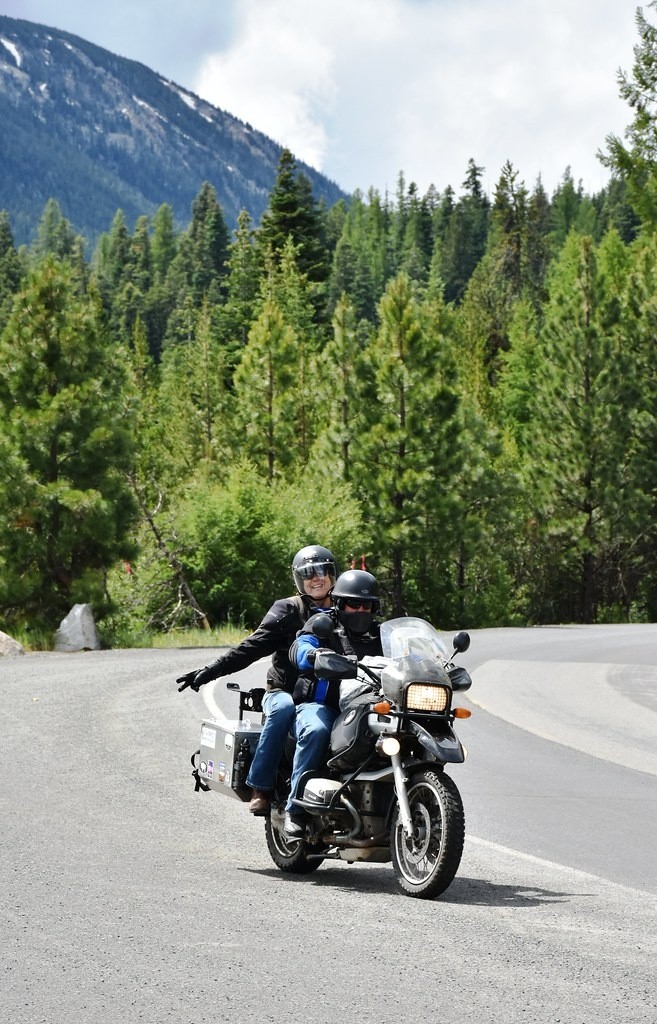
[[331, 570, 380, 600], [292, 545, 336, 568]]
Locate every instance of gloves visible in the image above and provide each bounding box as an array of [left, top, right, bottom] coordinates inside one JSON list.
[[175, 659, 222, 693]]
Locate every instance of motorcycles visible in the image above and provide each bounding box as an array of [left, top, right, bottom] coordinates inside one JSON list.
[[191, 617, 470, 899]]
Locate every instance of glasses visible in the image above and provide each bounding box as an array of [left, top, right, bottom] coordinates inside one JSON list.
[[297, 565, 331, 579], [344, 598, 373, 609]]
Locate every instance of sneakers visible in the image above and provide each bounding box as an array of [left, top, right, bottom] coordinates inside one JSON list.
[[284, 812, 306, 835], [249, 789, 271, 812]]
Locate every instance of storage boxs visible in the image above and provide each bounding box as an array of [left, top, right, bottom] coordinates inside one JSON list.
[[198, 718, 264, 803]]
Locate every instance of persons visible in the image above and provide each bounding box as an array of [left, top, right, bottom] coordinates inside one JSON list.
[[283, 569, 457, 836], [176, 545, 383, 813]]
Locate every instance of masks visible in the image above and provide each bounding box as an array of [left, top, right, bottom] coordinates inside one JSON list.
[[340, 611, 377, 632]]
